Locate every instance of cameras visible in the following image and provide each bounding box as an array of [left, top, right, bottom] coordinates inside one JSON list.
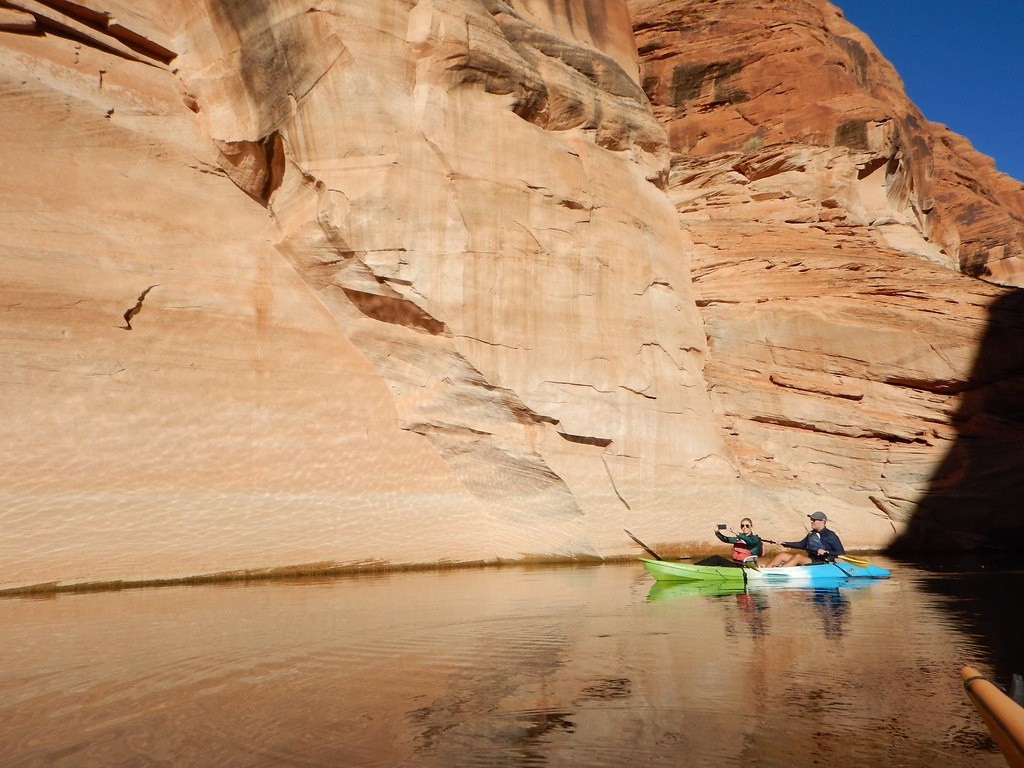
[[717, 524, 727, 529]]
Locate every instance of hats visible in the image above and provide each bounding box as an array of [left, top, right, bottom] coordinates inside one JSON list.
[[807, 512, 827, 522]]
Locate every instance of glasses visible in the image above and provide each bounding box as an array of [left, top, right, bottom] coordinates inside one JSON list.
[[741, 524, 751, 528]]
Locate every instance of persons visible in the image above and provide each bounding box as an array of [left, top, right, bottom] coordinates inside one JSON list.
[[758, 512, 845, 567], [693, 518, 760, 567]]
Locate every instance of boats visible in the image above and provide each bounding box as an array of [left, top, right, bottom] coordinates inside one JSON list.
[[645, 581, 744, 605], [638, 557, 892, 580]]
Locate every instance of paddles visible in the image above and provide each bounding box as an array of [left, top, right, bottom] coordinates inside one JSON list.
[[759, 538, 869, 568], [736, 562, 761, 572]]
